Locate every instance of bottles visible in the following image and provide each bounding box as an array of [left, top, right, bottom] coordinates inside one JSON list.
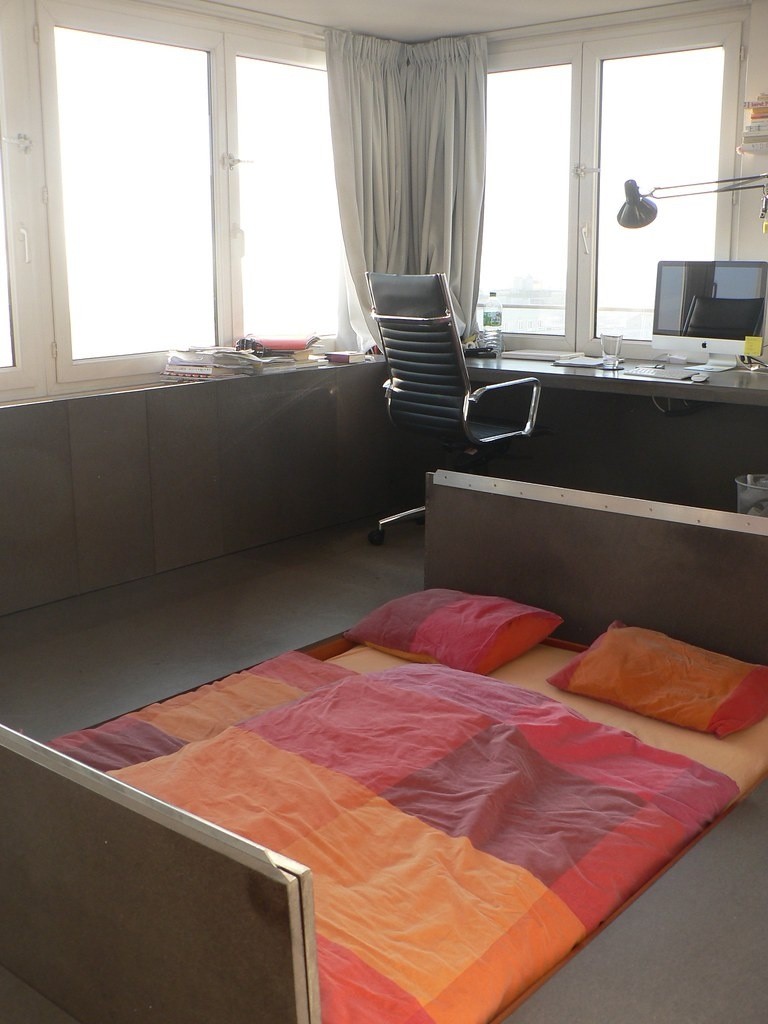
[[482, 292, 502, 358]]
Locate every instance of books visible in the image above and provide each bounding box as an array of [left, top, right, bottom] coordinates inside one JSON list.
[[159, 332, 371, 385], [736, 93, 767, 156]]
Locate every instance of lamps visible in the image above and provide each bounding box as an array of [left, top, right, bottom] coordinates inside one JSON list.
[[617, 173, 768, 229]]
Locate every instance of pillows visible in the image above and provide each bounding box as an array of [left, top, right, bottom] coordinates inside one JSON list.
[[341, 588, 565, 677], [546, 619, 767, 740]]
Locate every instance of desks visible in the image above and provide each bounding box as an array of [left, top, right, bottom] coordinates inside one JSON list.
[[460, 356, 768, 407]]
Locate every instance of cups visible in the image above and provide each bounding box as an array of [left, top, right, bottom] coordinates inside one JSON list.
[[600, 334, 623, 366]]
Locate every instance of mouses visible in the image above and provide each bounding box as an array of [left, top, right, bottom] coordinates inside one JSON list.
[[691, 375, 709, 382]]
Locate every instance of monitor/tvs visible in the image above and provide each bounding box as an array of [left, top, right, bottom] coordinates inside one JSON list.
[[653, 261, 768, 371]]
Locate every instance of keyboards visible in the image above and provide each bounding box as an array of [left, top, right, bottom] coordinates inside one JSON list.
[[623, 368, 700, 380]]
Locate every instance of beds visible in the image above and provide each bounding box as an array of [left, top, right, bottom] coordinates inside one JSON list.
[[0, 470, 767, 1023]]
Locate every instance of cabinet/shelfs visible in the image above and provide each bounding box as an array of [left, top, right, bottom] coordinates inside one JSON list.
[[0, 353, 387, 618]]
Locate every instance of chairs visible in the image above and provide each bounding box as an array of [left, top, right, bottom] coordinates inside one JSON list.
[[364, 269, 542, 545]]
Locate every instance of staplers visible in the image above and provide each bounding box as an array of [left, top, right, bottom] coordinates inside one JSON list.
[[464, 346, 497, 358]]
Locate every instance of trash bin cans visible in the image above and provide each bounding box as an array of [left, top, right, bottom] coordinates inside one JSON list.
[[734, 474, 768, 518]]
